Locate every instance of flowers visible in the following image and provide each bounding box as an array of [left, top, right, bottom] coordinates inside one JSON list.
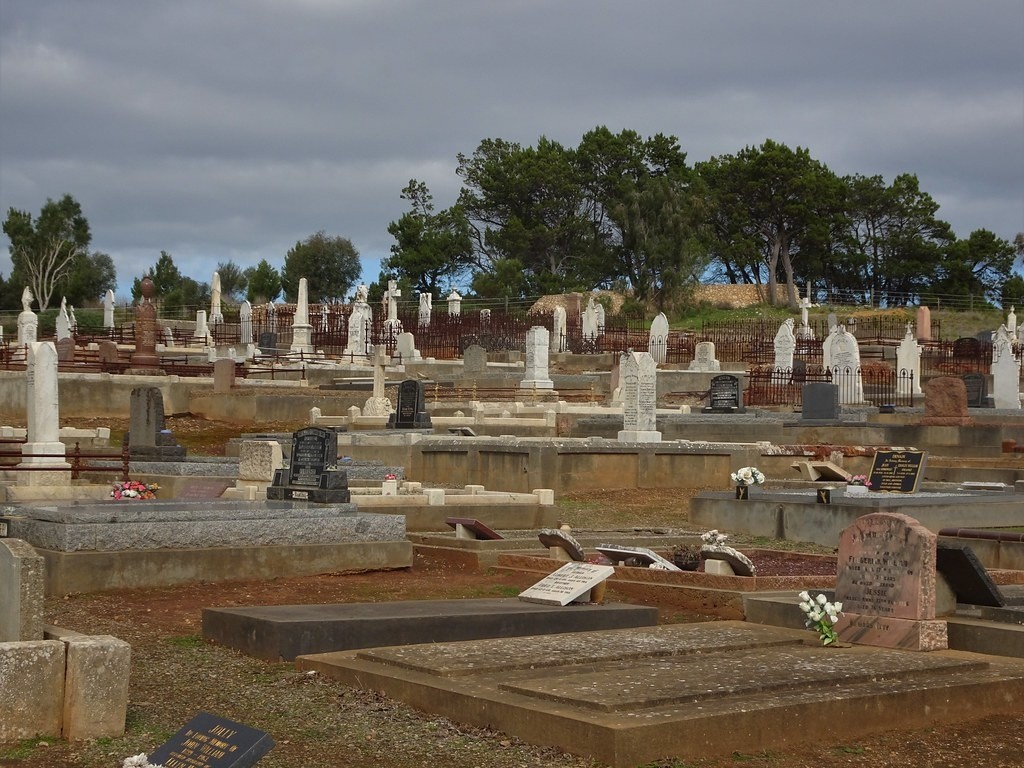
[[110, 482, 161, 499], [123, 752, 167, 768], [796, 589, 847, 646], [730, 466, 765, 486]]
